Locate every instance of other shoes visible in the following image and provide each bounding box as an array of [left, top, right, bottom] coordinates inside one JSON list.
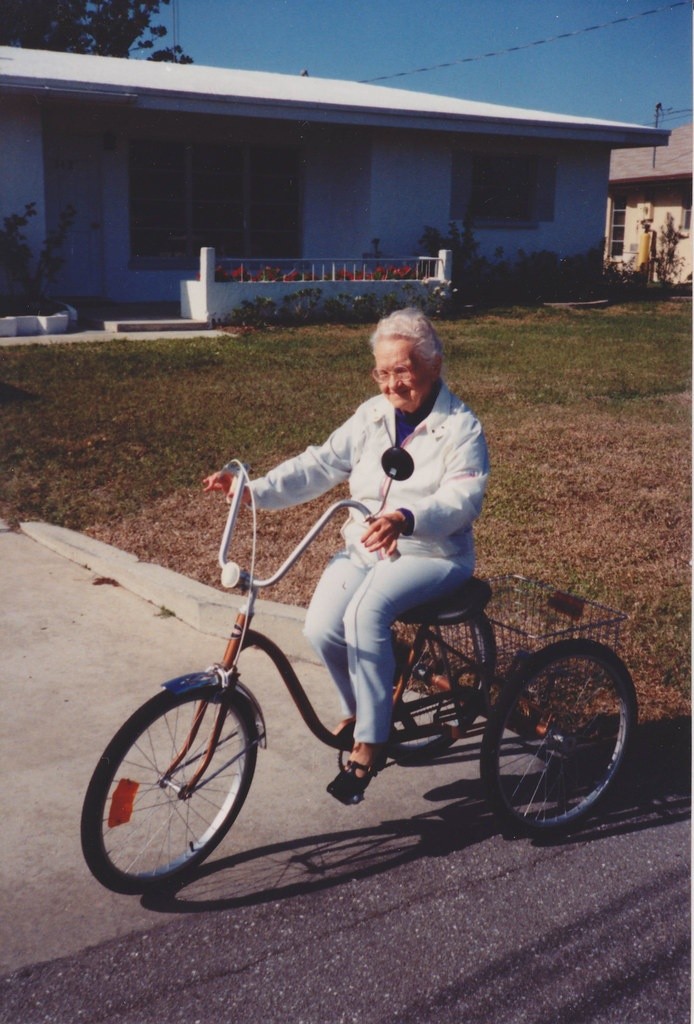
[[327, 760, 380, 801]]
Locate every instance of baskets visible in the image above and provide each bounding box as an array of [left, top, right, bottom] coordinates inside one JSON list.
[[389, 572, 627, 703]]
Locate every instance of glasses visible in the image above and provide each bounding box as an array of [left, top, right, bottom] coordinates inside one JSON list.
[[372, 365, 425, 383]]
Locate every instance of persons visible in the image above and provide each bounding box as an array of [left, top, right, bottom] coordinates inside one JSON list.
[[203, 309, 489, 806]]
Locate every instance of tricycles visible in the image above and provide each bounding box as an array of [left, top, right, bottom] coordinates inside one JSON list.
[[81, 446, 637, 901]]
